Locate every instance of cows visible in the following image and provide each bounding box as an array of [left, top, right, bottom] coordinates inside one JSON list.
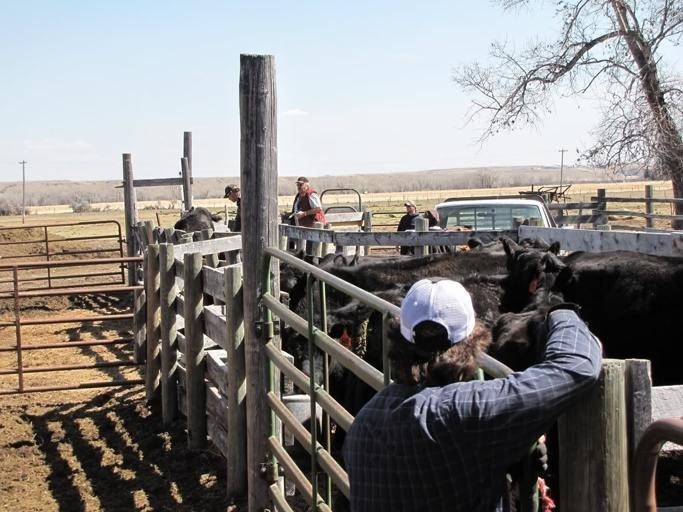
[[280, 236, 683, 512], [174, 206, 223, 242]]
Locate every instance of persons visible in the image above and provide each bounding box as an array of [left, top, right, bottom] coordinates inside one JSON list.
[[394, 201, 419, 255], [341, 277, 604, 511], [289, 176, 327, 255], [223, 184, 241, 232]]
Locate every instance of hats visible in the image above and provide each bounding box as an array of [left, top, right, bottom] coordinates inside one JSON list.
[[296, 177, 309, 183], [405, 201, 416, 207], [224, 183, 237, 198], [399, 276, 475, 345]]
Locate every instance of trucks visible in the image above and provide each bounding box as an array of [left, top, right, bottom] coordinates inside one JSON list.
[[428, 196, 560, 245]]
